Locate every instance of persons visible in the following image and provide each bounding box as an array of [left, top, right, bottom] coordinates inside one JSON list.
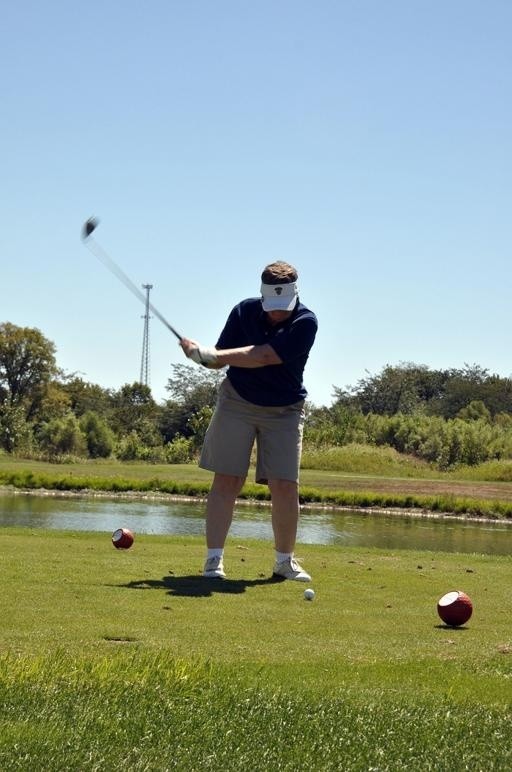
[[178, 259, 318, 582]]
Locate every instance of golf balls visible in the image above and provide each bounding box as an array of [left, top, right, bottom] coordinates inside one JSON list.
[[305, 589, 315, 599]]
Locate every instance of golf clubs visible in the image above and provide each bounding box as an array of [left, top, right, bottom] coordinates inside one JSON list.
[[82, 217, 184, 342]]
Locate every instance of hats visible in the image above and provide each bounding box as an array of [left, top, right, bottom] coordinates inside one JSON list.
[[260, 282, 299, 312]]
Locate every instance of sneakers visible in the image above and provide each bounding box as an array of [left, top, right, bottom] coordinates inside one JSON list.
[[273, 557, 312, 583], [203, 555, 226, 578]]
